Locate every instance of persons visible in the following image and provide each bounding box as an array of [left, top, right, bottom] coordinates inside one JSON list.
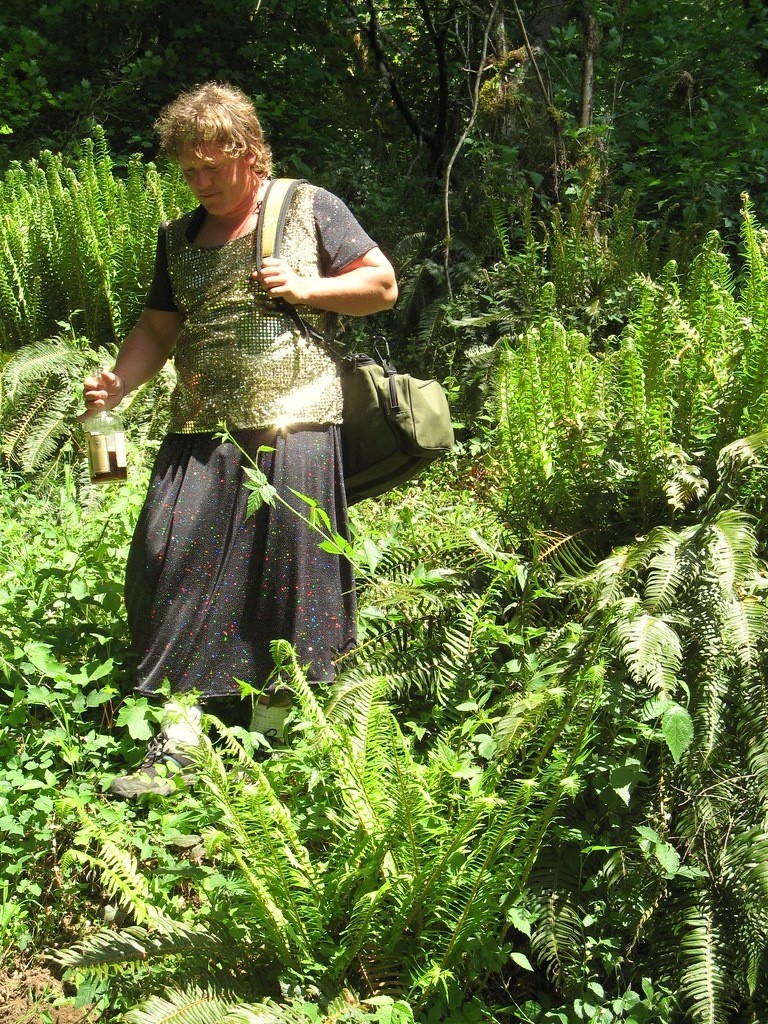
[[80, 78, 400, 803]]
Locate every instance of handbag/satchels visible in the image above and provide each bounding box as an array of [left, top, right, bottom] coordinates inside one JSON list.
[[342, 352, 455, 511]]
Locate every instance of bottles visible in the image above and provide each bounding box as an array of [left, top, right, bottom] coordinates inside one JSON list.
[[84, 366, 127, 484]]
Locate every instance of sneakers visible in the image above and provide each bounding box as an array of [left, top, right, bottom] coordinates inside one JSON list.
[[111, 731, 207, 799], [253, 734, 310, 803]]
